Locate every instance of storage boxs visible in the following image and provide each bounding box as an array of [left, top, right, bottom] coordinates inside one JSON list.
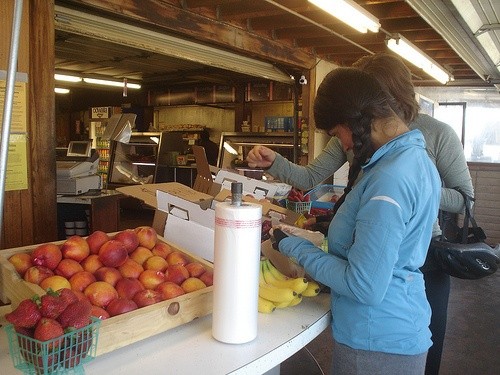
[[115, 145, 304, 265], [0, 227, 214, 358]]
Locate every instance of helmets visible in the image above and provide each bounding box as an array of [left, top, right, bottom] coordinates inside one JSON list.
[[430, 226, 500, 279]]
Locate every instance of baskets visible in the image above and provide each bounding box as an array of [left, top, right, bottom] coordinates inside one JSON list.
[[3, 314, 101, 375]]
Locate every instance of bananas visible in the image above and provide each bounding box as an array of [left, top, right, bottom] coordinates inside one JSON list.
[[258, 256, 321, 312]]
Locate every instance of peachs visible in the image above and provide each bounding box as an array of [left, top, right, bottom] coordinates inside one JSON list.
[[8, 225, 213, 320]]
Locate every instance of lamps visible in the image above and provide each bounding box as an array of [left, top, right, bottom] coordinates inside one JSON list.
[[53, 68, 142, 95], [308, 0, 382, 34], [384, 32, 455, 86]]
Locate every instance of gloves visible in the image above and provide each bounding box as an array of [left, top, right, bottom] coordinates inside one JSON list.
[[271, 228, 289, 251]]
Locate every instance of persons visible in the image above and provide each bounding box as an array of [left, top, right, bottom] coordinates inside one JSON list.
[[273, 68, 441, 375], [246, 54, 474, 374], [197, 130, 219, 166]]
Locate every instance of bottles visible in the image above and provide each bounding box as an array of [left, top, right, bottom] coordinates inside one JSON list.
[[94, 124, 113, 190]]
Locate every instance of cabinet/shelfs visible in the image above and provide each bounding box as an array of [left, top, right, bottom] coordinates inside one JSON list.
[[58, 189, 121, 239], [106, 131, 209, 222]]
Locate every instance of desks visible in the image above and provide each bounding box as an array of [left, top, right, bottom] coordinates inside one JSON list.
[[0, 287, 331, 375]]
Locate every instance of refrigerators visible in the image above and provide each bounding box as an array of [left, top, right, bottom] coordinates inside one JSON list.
[[90, 106, 126, 176]]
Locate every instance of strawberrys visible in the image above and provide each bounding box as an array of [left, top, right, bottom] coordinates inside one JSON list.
[[5, 288, 92, 372], [287, 188, 310, 202], [310, 197, 336, 215]]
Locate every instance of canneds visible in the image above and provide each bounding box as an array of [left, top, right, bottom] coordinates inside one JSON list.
[[96, 126, 111, 183]]
